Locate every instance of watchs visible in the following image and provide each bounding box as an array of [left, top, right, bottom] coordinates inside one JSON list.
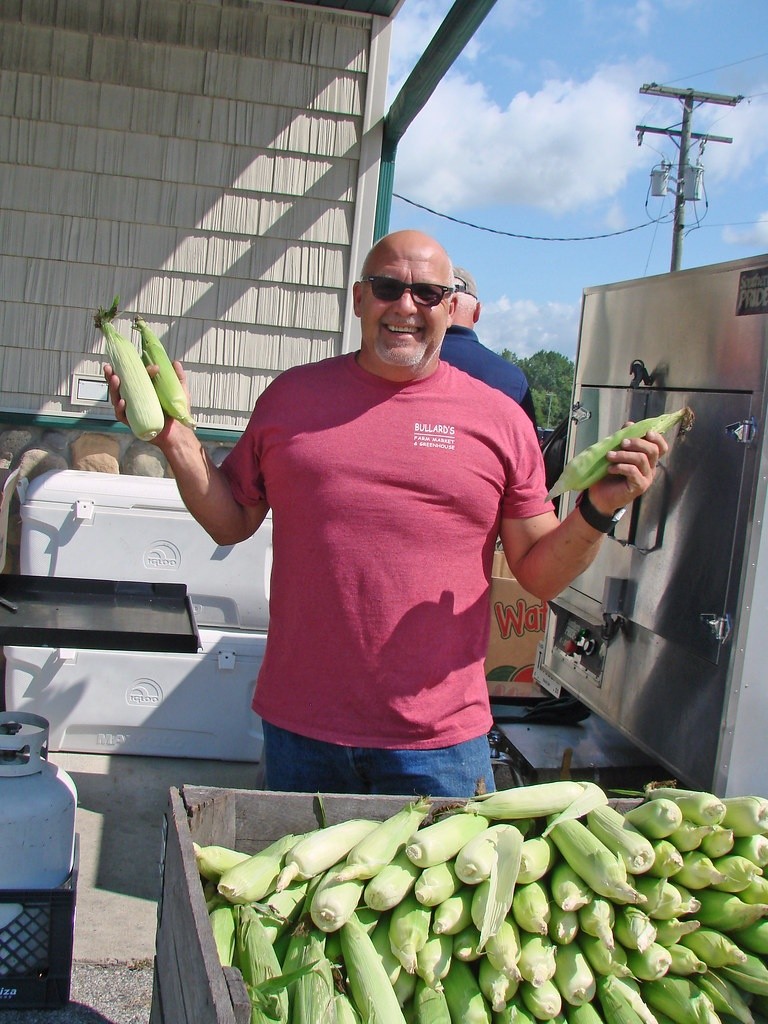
[[575, 486, 626, 534]]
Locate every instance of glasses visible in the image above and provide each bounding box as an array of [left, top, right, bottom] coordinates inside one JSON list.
[[362, 276, 453, 307]]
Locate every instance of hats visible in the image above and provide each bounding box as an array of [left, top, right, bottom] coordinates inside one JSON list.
[[453, 266, 478, 300]]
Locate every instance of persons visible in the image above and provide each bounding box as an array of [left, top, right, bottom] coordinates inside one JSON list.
[[104, 230, 669, 799]]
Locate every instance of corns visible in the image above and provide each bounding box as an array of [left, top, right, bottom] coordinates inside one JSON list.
[[188, 781, 768, 1024], [544, 407, 691, 502], [94, 294, 197, 442]]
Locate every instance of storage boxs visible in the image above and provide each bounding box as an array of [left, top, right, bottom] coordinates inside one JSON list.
[[0, 833, 80, 1008], [484, 551, 548, 683], [148, 784, 645, 1024], [3, 468, 273, 761]]
